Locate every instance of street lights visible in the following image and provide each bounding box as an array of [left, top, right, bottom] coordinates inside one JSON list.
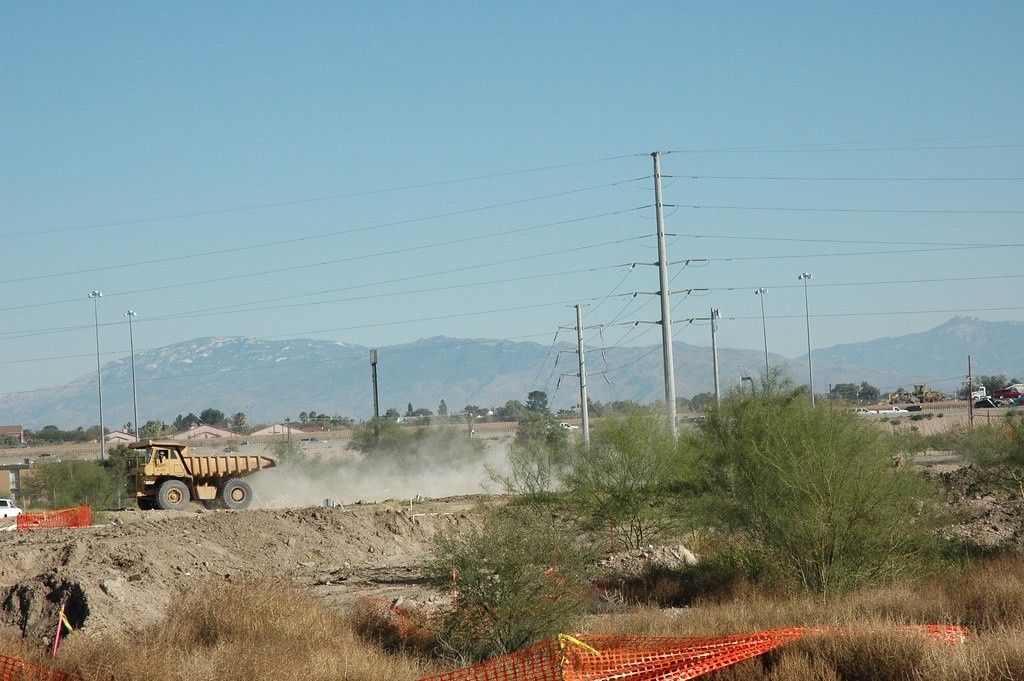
[[755, 288, 770, 395], [798, 272, 815, 409], [124, 310, 139, 442], [88, 289, 107, 461]]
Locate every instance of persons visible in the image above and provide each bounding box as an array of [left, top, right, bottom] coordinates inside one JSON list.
[[159, 452, 166, 459]]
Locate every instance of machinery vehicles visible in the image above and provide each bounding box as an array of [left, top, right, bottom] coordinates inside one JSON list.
[[126, 437, 279, 510]]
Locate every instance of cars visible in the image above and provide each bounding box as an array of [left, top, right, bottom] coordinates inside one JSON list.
[[0, 498, 23, 519], [560, 423, 579, 430], [847, 406, 909, 414], [972, 385, 1024, 408]]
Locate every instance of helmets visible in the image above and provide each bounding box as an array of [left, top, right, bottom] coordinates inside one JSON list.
[[160, 451, 164, 454]]
